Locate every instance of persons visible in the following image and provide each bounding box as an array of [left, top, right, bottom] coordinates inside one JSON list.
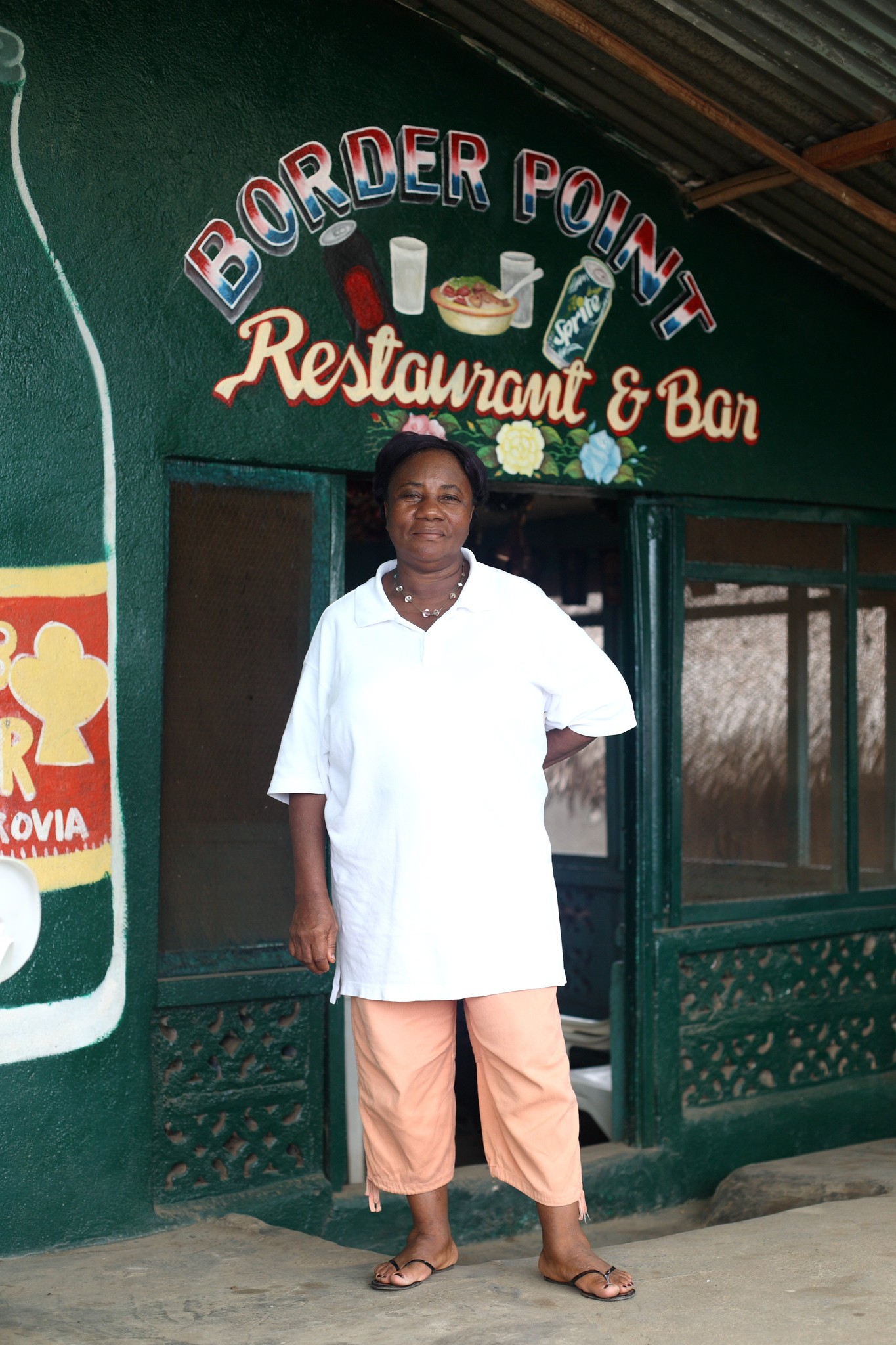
[[266, 432, 639, 1302]]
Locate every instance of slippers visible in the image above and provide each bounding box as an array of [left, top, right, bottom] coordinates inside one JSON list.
[[371, 1259, 455, 1291], [541, 1265, 637, 1299]]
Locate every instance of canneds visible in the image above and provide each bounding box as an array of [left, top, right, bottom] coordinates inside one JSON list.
[[318, 220, 405, 354], [544, 255, 616, 370]]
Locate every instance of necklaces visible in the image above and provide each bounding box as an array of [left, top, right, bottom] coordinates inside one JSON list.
[[392, 561, 466, 619]]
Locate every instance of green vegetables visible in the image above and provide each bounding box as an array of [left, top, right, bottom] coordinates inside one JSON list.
[[450, 274, 498, 292]]
